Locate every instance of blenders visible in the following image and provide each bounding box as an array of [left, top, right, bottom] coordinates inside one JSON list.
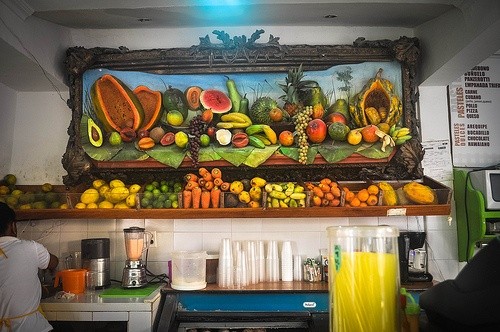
[[121, 227, 153, 288]]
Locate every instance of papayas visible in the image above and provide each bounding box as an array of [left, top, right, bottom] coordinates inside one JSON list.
[[378, 181, 435, 206]]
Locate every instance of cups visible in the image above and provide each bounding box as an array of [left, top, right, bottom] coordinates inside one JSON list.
[[217, 237, 303, 288], [303, 265, 322, 282], [86, 271, 95, 294], [168, 261, 172, 283], [72, 251, 81, 268]]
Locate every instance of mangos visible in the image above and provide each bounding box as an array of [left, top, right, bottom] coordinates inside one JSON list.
[[230, 177, 266, 208], [76, 178, 141, 209]]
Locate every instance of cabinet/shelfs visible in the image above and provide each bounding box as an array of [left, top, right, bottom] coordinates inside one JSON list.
[[452, 168, 500, 261]]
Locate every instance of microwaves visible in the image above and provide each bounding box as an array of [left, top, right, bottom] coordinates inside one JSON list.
[[468, 169, 500, 212]]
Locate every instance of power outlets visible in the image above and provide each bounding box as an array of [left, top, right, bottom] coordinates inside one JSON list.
[[148, 231, 157, 248]]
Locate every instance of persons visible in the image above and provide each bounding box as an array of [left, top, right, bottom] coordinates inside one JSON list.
[[0, 201, 59, 332], [401, 239, 500, 332]]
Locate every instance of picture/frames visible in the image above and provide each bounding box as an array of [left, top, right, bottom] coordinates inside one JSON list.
[[61, 27, 422, 189]]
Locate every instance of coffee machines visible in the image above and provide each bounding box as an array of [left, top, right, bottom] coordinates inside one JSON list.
[[398, 231, 433, 288]]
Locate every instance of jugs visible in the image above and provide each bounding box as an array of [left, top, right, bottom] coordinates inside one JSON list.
[[54, 269, 88, 296]]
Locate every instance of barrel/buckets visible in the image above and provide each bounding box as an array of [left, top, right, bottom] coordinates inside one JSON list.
[[206, 254, 219, 284], [81, 238, 111, 289], [326, 225, 401, 332], [170, 250, 206, 291]]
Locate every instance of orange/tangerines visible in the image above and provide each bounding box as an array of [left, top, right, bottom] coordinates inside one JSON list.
[[342, 185, 380, 207], [140, 179, 181, 209], [0, 173, 68, 211]]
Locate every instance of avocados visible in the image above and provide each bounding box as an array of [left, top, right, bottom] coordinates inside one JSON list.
[[224, 178, 251, 209]]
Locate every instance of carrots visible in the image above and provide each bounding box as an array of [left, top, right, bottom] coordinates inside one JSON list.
[[182, 167, 223, 208], [221, 182, 230, 191]]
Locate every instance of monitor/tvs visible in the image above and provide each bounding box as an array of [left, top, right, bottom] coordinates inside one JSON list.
[[470, 169, 500, 211]]
